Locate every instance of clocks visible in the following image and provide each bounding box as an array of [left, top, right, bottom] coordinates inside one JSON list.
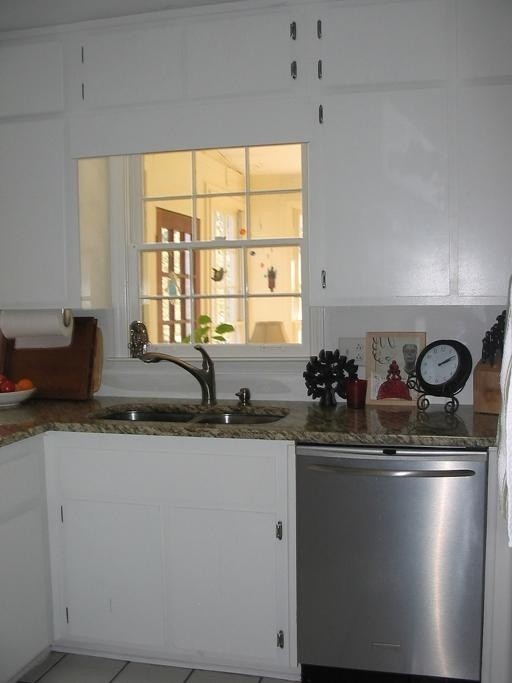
[[416, 338, 473, 397]]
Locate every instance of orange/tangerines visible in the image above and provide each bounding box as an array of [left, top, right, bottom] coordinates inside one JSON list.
[[15, 378, 32, 392]]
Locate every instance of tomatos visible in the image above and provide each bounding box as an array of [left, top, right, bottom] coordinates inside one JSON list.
[[0, 373, 15, 392]]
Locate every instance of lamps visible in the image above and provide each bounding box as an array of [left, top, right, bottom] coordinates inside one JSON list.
[[247, 321, 289, 344]]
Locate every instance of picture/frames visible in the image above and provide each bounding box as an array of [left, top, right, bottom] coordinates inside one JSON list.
[[364, 331, 426, 406]]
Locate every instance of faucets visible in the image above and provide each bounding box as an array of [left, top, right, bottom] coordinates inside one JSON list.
[[138, 345, 217, 406]]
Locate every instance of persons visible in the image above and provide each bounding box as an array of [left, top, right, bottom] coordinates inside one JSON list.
[[398, 342, 417, 380]]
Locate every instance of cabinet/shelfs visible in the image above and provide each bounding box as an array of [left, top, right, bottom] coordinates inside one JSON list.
[[0, 20, 113, 310], [308, 0, 512, 308], [72, 0, 307, 157]]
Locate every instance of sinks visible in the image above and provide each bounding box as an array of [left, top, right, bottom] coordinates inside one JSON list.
[[96, 409, 196, 423], [194, 411, 285, 425]]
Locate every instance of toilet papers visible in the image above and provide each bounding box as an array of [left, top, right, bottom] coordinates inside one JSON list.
[[0, 307, 74, 350]]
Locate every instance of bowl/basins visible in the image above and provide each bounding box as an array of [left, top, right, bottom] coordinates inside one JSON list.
[[0, 387, 37, 408]]
